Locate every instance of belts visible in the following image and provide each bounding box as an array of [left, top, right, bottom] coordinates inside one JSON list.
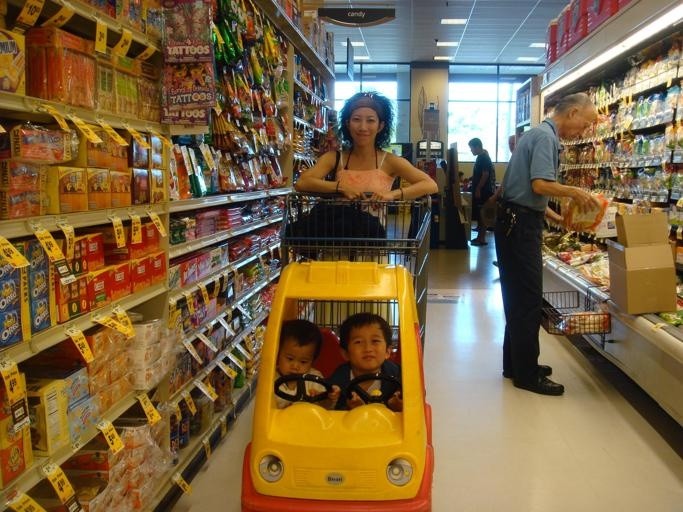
[[499, 198, 545, 215]]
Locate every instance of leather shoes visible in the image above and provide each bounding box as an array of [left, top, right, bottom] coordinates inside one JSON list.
[[513, 375, 563, 396], [503, 365, 551, 378]]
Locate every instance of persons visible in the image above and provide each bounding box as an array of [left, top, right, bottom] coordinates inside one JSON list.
[[272, 318, 340, 411], [293, 89, 438, 264], [487, 135, 515, 206], [490, 93, 608, 394], [325, 311, 403, 412], [467, 137, 495, 246]]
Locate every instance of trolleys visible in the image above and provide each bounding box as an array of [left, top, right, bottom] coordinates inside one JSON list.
[[274, 180, 437, 364]]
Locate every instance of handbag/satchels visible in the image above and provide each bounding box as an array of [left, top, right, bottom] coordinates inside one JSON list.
[[285, 201, 386, 247], [480, 199, 498, 228]]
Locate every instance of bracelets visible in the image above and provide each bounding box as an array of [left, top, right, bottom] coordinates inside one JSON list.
[[335, 180, 340, 193], [399, 187, 403, 201]]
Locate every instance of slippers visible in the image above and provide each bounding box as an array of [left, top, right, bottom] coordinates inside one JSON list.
[[471, 239, 488, 245]]
